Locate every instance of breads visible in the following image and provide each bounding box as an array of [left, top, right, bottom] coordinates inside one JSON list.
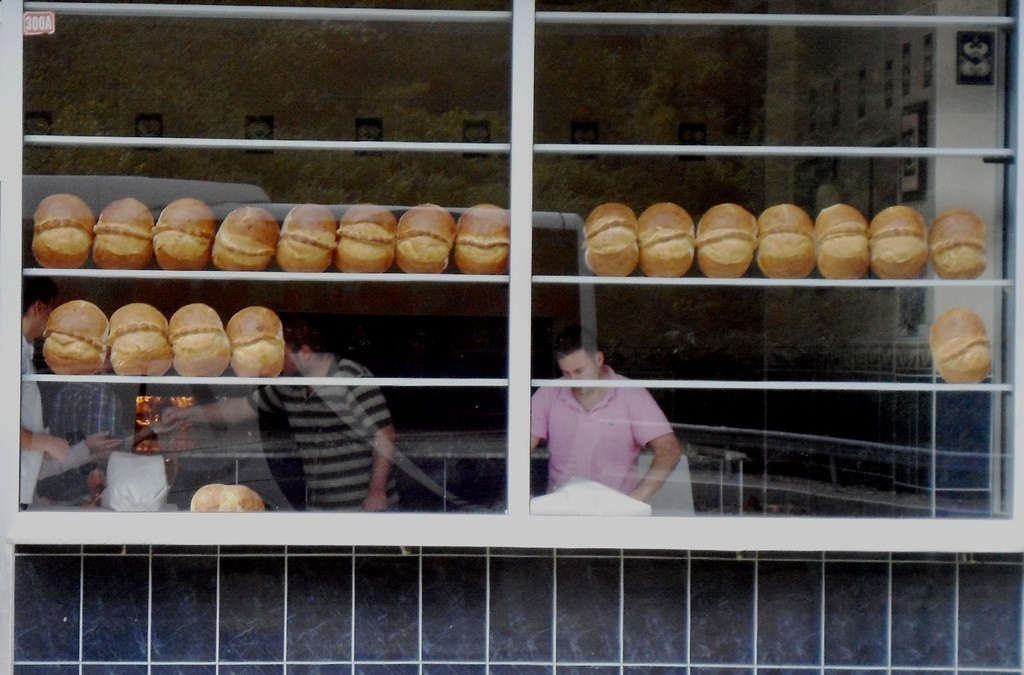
[[190, 483, 265, 512], [168, 303, 230, 376], [930, 307, 993, 385], [43, 300, 109, 375], [109, 303, 173, 377], [33, 194, 510, 276], [583, 204, 986, 280], [227, 306, 285, 378]]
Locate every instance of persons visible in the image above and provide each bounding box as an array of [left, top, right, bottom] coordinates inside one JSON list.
[[162, 321, 400, 512], [20, 275, 70, 508], [49, 381, 192, 502], [531, 325, 682, 503]]
